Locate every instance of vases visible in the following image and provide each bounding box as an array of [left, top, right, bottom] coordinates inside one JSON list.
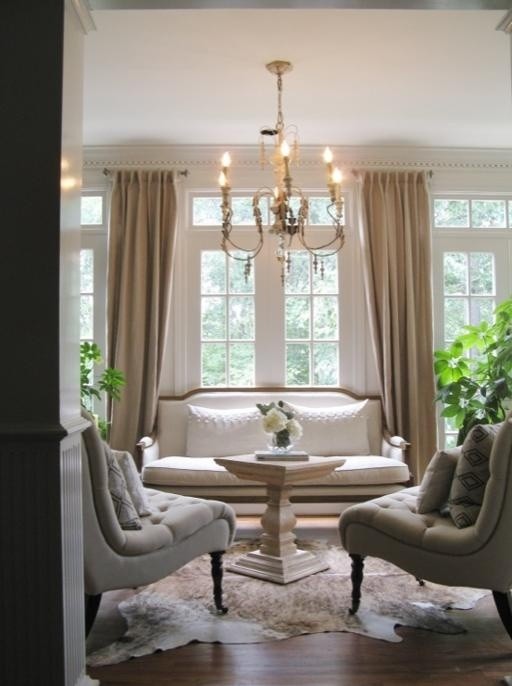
[[266, 432, 291, 454]]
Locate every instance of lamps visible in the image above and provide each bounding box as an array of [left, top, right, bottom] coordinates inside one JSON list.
[[218, 61, 348, 288]]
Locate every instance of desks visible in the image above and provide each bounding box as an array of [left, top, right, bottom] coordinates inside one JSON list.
[[214, 454, 346, 585]]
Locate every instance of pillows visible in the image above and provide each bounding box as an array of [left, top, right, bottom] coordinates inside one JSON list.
[[185, 404, 269, 457], [416, 445, 463, 513], [287, 399, 381, 456], [112, 449, 151, 517], [440, 422, 503, 529], [102, 441, 142, 530]]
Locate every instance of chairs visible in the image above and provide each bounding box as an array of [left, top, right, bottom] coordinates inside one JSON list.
[[81, 405, 237, 639], [338, 412, 512, 639]]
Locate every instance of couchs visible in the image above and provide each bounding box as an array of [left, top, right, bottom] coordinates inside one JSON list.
[[136, 387, 412, 515]]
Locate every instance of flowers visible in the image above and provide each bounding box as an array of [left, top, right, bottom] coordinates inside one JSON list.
[[257, 400, 302, 449]]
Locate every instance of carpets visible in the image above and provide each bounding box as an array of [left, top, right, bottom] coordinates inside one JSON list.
[[86, 537, 494, 668]]
